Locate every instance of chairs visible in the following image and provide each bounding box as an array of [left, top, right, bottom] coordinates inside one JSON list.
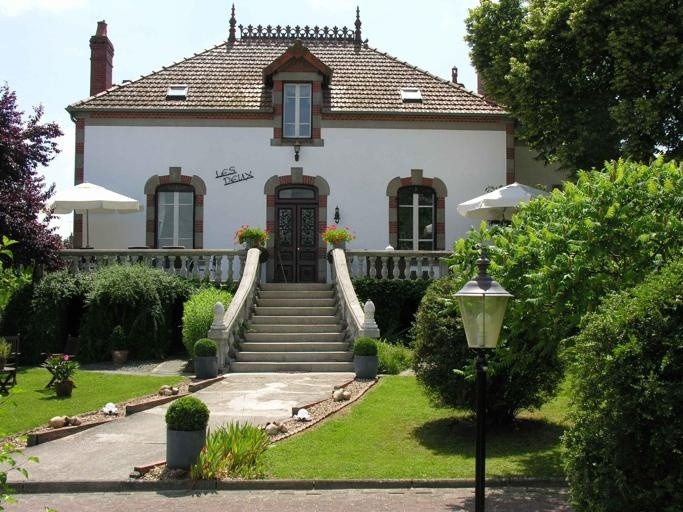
[[3, 333, 21, 388], [127, 245, 193, 274], [41, 334, 81, 391]]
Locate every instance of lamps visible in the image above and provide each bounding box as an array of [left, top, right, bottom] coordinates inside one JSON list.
[[292, 140, 301, 161], [334, 205, 341, 224]]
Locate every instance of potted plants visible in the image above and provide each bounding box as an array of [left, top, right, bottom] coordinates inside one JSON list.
[[165, 396, 211, 469], [0, 337, 12, 369], [351, 337, 379, 379], [192, 338, 219, 379], [107, 325, 129, 366]]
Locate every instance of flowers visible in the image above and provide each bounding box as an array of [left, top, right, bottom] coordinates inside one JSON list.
[[234, 224, 271, 246], [319, 223, 356, 244], [40, 353, 80, 381]]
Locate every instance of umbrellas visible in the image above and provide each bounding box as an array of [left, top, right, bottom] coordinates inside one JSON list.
[[457, 181, 551, 221], [46, 181, 139, 248]]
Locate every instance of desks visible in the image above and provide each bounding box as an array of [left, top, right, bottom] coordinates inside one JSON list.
[[0, 367, 16, 395]]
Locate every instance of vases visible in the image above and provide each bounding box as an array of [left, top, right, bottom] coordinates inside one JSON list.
[[55, 381, 74, 398], [333, 241, 346, 248], [246, 238, 260, 249]]
